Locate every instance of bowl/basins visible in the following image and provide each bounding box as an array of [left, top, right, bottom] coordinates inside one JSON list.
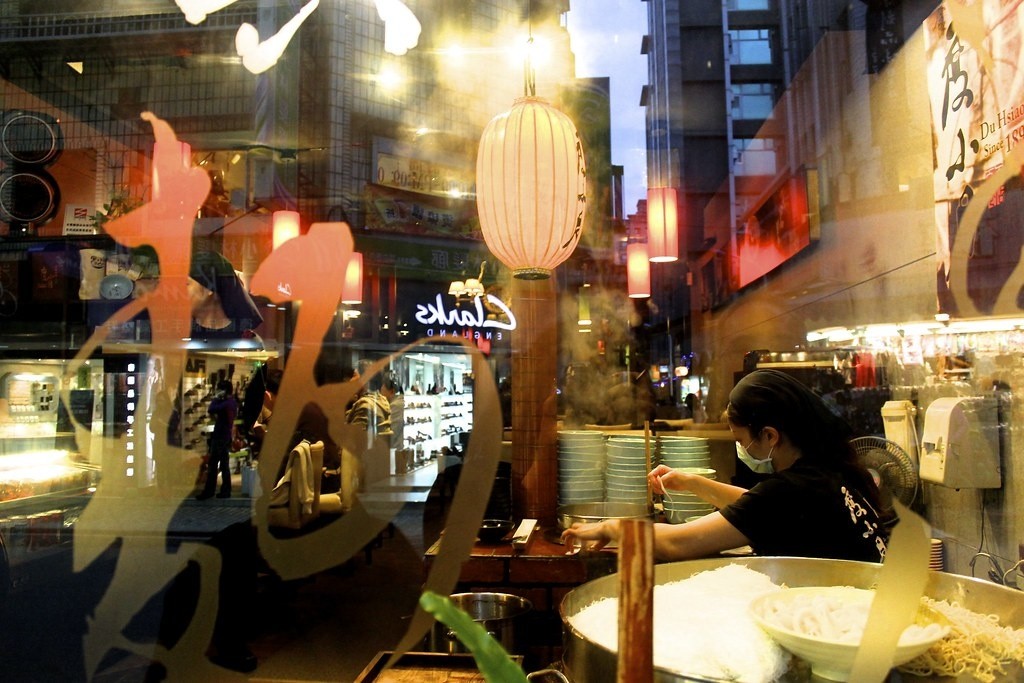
[[477, 520, 515, 545], [99, 273, 133, 300], [751, 587, 951, 683], [557, 431, 717, 525]]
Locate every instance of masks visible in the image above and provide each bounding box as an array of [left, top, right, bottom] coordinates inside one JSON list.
[[736, 437, 775, 474]]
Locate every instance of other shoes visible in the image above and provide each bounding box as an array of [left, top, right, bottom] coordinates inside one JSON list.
[[216, 492, 232, 499], [195, 491, 215, 500]]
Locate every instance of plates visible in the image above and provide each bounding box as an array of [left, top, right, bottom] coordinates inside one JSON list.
[[928, 539, 943, 571]]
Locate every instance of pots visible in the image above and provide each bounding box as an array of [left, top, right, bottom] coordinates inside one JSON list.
[[554, 501, 665, 536], [557, 555, 1024, 683]]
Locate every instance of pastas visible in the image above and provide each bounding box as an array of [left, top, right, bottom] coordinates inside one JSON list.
[[897, 595, 1024, 678]]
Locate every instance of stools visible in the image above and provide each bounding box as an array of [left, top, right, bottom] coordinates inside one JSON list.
[[252, 439, 365, 528]]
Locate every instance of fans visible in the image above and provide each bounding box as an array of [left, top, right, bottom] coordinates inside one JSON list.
[[849, 436, 917, 527]]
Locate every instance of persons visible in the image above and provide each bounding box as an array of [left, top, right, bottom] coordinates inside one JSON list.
[[561, 369, 889, 563], [199, 194, 243, 255], [120, 246, 268, 432], [684, 393, 707, 423], [195, 379, 239, 500], [252, 359, 406, 489], [91, 353, 432, 682]]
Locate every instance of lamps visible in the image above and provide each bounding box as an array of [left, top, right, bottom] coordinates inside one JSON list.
[[341, 251, 363, 305], [448, 279, 485, 307], [578, 266, 592, 333], [272, 151, 301, 251], [627, 243, 651, 299], [648, 187, 678, 262]]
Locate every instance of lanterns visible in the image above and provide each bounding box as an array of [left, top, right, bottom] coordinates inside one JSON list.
[[475, 94, 588, 281]]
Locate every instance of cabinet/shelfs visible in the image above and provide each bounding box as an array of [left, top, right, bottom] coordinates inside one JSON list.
[[389, 358, 474, 476], [0, 359, 105, 566]]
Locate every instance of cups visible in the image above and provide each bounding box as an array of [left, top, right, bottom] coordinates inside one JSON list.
[[429, 592, 535, 655], [880, 399, 919, 487]]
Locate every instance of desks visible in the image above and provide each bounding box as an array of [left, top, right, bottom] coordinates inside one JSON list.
[[0, 504, 258, 673], [423, 514, 665, 663]]
